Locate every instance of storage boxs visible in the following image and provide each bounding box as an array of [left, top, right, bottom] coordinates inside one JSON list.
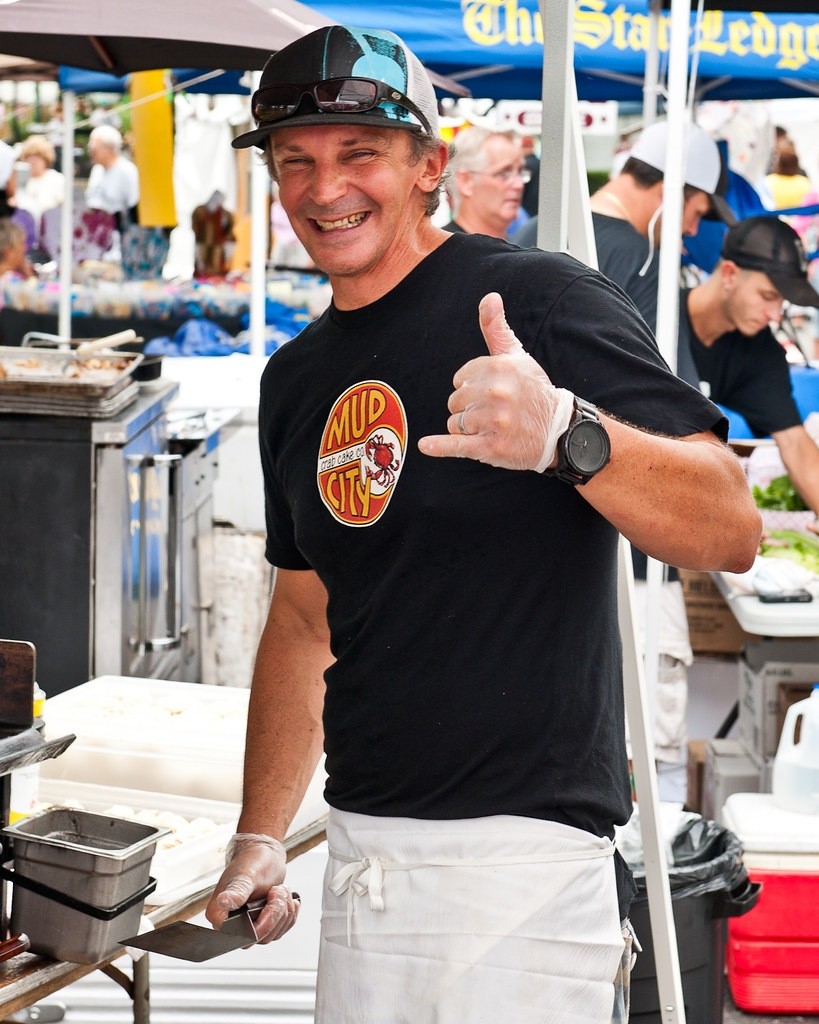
[[36, 675, 328, 805], [720, 792, 819, 1013], [687, 639, 818, 821], [10, 772, 305, 905], [2, 807, 171, 973], [677, 567, 819, 654]]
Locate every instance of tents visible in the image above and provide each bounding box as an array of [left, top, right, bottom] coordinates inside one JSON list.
[[56, 0, 819, 357]]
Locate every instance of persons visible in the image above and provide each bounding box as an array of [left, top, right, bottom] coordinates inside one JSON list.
[[205, 30, 771, 1024], [0, 126, 819, 813]]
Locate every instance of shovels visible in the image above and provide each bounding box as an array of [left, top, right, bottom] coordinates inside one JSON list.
[[118, 892, 301, 963]]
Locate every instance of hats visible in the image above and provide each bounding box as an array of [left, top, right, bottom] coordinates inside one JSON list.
[[630, 118, 740, 227], [232, 23, 439, 152], [720, 216, 819, 309]]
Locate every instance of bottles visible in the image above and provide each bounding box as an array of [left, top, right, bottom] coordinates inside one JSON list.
[[771, 684, 819, 815]]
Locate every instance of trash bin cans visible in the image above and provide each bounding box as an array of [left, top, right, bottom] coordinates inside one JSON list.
[[629, 816, 763, 1024]]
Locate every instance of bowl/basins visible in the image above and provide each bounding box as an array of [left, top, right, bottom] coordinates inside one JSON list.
[[127, 351, 164, 381]]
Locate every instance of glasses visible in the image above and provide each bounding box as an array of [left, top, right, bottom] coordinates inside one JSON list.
[[251, 76, 434, 138], [466, 169, 532, 183]]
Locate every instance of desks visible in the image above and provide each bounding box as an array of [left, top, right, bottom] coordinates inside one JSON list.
[[3, 269, 333, 350]]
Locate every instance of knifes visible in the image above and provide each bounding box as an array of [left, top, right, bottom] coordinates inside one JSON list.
[[117, 892, 302, 963]]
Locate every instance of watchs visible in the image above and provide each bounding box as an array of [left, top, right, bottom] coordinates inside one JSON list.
[[541, 397, 612, 487]]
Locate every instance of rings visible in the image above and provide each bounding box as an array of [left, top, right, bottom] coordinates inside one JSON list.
[[457, 411, 470, 434]]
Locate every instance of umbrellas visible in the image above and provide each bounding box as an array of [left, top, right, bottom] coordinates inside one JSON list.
[[0, 0, 469, 108]]
